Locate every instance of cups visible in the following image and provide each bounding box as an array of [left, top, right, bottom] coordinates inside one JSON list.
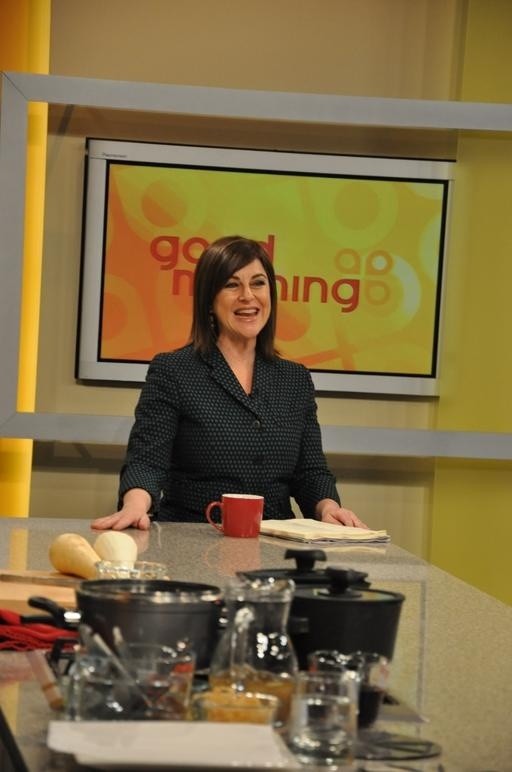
[[206, 493, 265, 538], [95, 558, 167, 581], [210, 571, 300, 724], [70, 639, 197, 720], [283, 669, 362, 767], [199, 686, 281, 725], [308, 646, 392, 729]]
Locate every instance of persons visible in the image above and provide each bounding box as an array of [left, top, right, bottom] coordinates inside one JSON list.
[[91, 235, 373, 532]]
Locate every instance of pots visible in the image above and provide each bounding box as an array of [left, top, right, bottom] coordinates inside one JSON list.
[[27, 579, 221, 672], [233, 542, 408, 672]]
